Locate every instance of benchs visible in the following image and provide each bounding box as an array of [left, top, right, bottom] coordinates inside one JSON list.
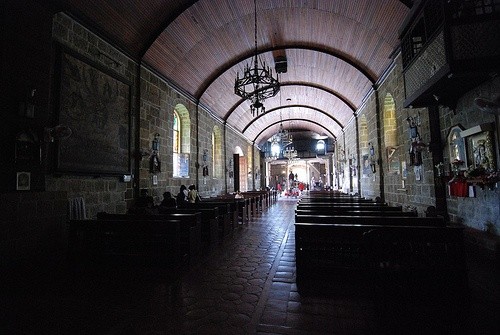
[[294, 189, 471, 309], [69, 190, 277, 283]]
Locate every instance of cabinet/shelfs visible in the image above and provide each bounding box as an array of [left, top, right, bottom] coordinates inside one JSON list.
[[396, 0, 500, 109]]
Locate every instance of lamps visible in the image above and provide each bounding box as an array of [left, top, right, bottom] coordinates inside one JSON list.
[[234, 0, 281, 118], [271, 85, 300, 164]]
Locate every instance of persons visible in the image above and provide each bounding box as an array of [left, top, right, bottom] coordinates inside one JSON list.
[[235, 190, 244, 199], [157, 192, 176, 211], [171, 184, 204, 208], [275, 170, 324, 197], [135, 190, 154, 211]]
